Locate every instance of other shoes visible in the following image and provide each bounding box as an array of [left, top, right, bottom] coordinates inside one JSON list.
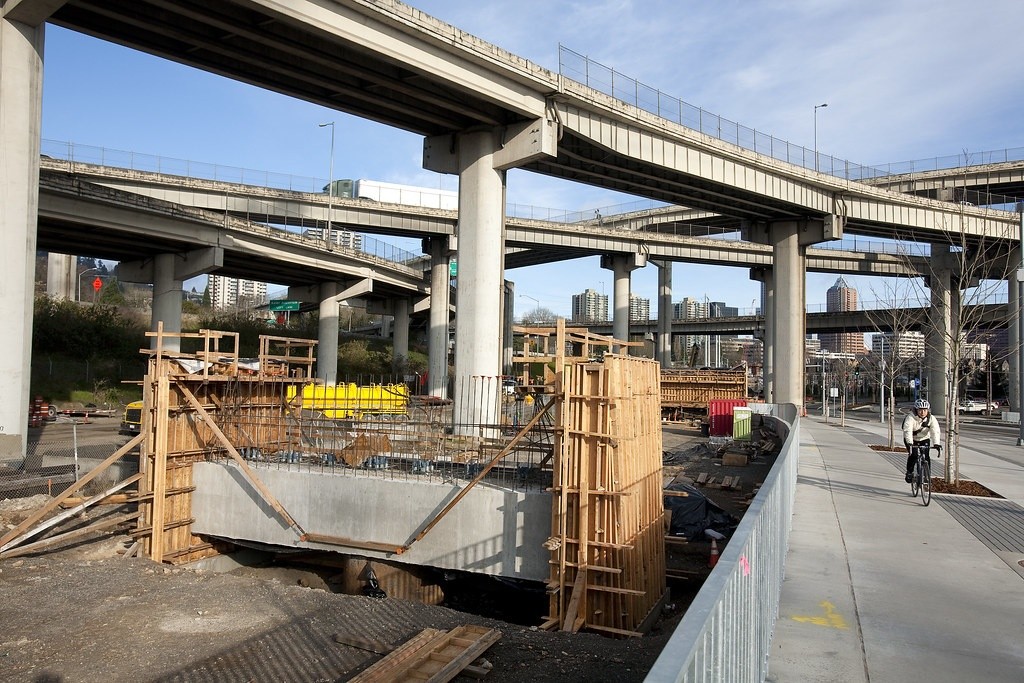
[[923, 477, 929, 484], [904, 473, 913, 484]]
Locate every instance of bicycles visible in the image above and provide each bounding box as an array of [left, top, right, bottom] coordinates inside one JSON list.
[[906, 443, 944, 506]]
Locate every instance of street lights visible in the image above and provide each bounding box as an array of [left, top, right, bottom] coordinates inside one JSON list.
[[79, 268, 97, 305], [520, 294, 540, 355], [814, 103, 828, 172], [599, 281, 604, 321], [319, 121, 334, 252]]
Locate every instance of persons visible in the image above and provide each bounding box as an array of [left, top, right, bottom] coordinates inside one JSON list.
[[903, 399, 941, 492]]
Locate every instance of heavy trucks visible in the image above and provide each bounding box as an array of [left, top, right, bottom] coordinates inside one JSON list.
[[118, 379, 409, 435], [324, 178, 459, 211]]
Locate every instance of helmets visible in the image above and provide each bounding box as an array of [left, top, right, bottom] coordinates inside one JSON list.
[[914, 399, 931, 410]]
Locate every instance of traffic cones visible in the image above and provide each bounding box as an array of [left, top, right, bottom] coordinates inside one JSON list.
[[709, 538, 721, 569]]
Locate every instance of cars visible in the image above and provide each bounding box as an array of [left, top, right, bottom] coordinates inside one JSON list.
[[503, 380, 518, 396]]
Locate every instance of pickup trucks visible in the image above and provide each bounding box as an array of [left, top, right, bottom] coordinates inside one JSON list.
[[955, 399, 999, 416]]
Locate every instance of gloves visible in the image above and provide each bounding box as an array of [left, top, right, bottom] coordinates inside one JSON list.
[[908, 443, 913, 450], [934, 444, 940, 449]]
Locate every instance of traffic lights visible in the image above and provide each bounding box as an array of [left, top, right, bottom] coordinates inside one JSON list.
[[921, 393, 925, 398], [855, 367, 860, 375], [916, 384, 921, 391], [922, 377, 927, 387]]
